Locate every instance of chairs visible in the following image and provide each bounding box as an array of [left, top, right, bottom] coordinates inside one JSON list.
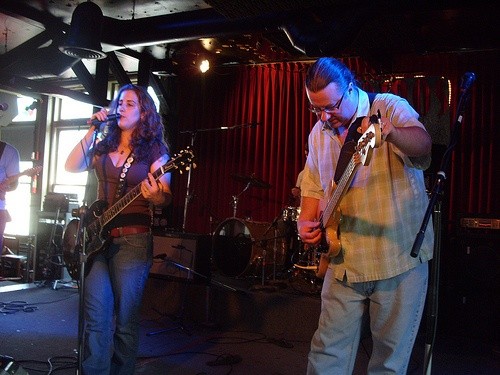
[[0, 235, 36, 278]]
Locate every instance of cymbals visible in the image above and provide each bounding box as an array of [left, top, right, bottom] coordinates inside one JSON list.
[[231, 176, 269, 188]]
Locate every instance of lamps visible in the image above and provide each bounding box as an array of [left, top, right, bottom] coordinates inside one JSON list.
[[188, 52, 210, 75]]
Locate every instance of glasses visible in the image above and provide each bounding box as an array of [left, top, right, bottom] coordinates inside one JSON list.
[[309, 84, 349, 114]]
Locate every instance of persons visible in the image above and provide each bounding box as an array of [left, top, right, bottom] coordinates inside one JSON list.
[[297, 57, 435, 375], [0, 141, 20, 257], [65, 84, 172, 375]]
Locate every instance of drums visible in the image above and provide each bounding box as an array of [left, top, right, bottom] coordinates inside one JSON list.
[[210, 217, 286, 274]]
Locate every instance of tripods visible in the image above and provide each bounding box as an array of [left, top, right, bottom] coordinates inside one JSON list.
[[240, 197, 296, 293]]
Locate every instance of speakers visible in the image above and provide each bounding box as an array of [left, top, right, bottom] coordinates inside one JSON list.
[[448, 237, 500, 351], [148, 231, 210, 283]]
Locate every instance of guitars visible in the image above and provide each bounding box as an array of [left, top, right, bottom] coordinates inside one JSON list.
[[298, 122, 382, 277], [63, 148, 197, 278]]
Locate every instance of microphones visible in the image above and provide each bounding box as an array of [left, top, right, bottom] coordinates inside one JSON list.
[[86, 113, 122, 125], [455, 72, 475, 124]]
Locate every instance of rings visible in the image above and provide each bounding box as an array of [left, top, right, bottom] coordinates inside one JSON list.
[[147, 185, 151, 190]]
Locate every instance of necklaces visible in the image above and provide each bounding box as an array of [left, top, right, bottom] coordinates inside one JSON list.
[[119, 141, 130, 155]]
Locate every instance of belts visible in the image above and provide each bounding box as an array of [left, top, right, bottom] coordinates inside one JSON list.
[[107, 224, 152, 238]]
